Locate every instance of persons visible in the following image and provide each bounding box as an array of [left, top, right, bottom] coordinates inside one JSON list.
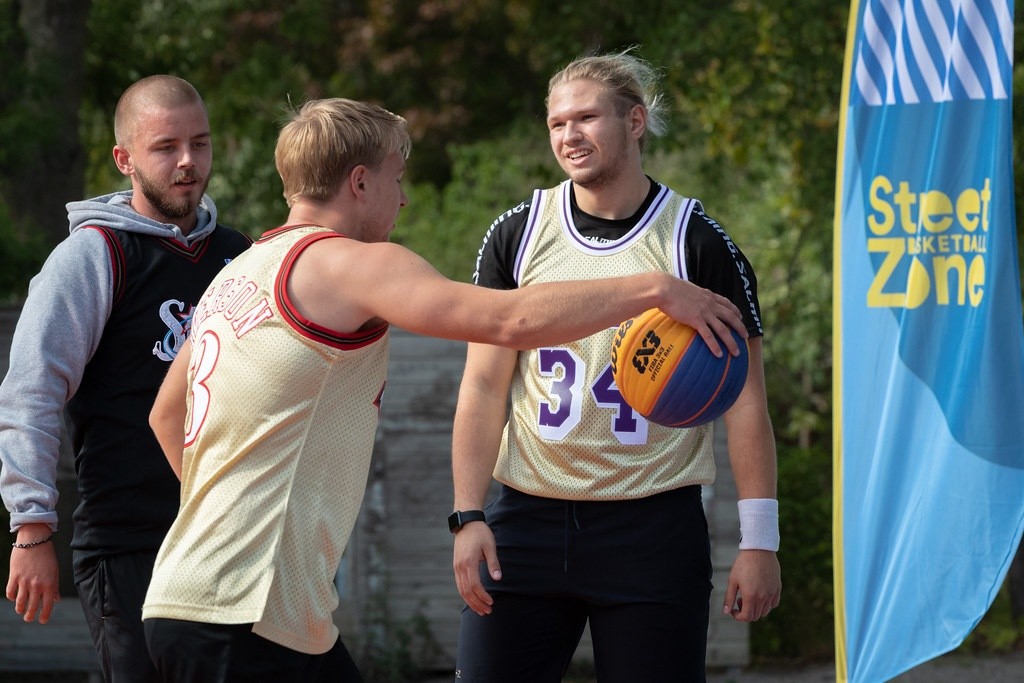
[[447, 46, 782, 683], [0, 75, 253, 683], [142, 91, 749, 683]]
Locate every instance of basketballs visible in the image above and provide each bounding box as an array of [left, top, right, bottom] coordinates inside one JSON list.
[[609, 304, 751, 430]]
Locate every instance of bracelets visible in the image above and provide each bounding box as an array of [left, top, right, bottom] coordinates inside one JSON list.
[[738, 499, 780, 552], [12, 536, 54, 548]]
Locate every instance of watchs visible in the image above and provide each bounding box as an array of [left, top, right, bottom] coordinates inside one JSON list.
[[448, 510, 486, 534]]
